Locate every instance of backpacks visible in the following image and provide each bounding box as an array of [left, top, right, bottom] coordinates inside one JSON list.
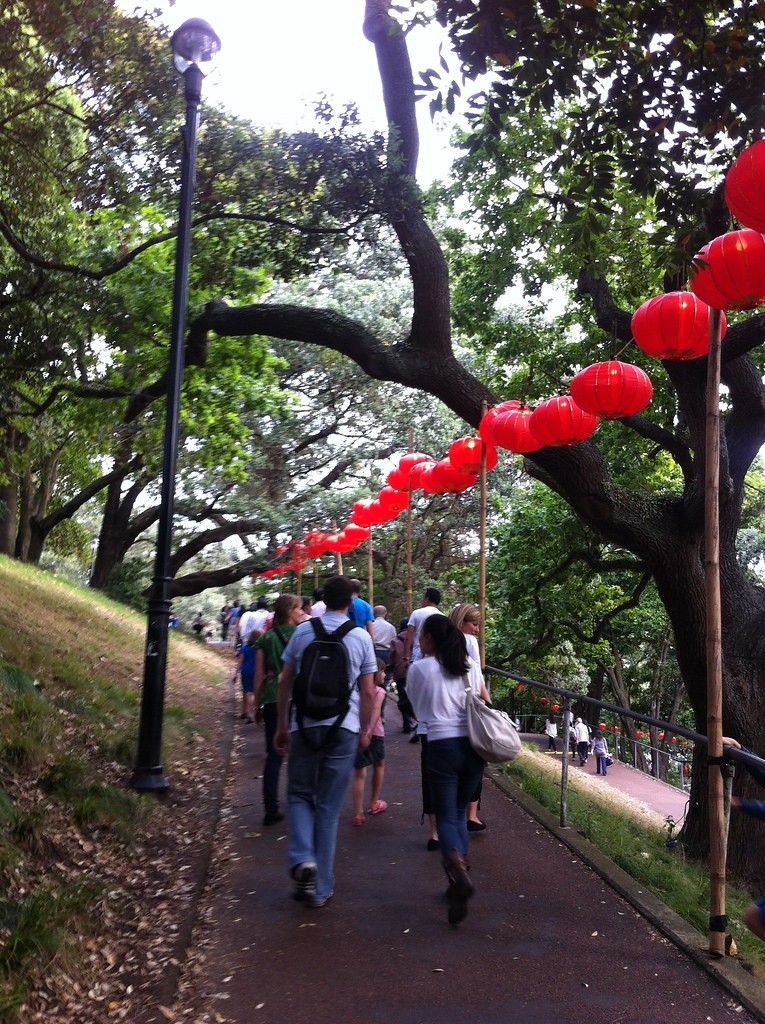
[[299, 619, 358, 721]]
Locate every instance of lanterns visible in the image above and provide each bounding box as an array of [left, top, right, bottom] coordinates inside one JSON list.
[[689, 229, 765, 311], [658, 733, 676, 743], [725, 139, 765, 235], [257, 437, 498, 579], [518, 685, 559, 711], [480, 395, 600, 453], [570, 361, 653, 419], [631, 292, 727, 361], [599, 723, 618, 732], [637, 731, 650, 736]]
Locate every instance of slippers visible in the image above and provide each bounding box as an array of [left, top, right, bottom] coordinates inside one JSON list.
[[351, 815, 365, 826], [367, 800, 388, 814]]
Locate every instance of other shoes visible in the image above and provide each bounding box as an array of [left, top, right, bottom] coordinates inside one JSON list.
[[288, 863, 317, 903], [440, 860, 475, 925], [467, 819, 486, 832], [428, 838, 438, 851], [262, 810, 285, 824], [408, 722, 422, 743], [307, 889, 334, 905]]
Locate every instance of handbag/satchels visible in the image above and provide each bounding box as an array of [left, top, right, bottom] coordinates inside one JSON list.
[[605, 757, 614, 766], [466, 692, 522, 761]]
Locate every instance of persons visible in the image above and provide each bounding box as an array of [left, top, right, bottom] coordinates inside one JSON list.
[[405, 614, 492, 926], [591, 730, 608, 776], [675, 753, 685, 771], [546, 714, 558, 753], [170, 612, 179, 627], [353, 657, 388, 826], [416, 603, 487, 851], [222, 595, 275, 723], [347, 579, 443, 743], [274, 574, 378, 907], [562, 712, 592, 765], [299, 588, 326, 622], [192, 612, 214, 642], [722, 737, 765, 942], [251, 594, 303, 825]]
